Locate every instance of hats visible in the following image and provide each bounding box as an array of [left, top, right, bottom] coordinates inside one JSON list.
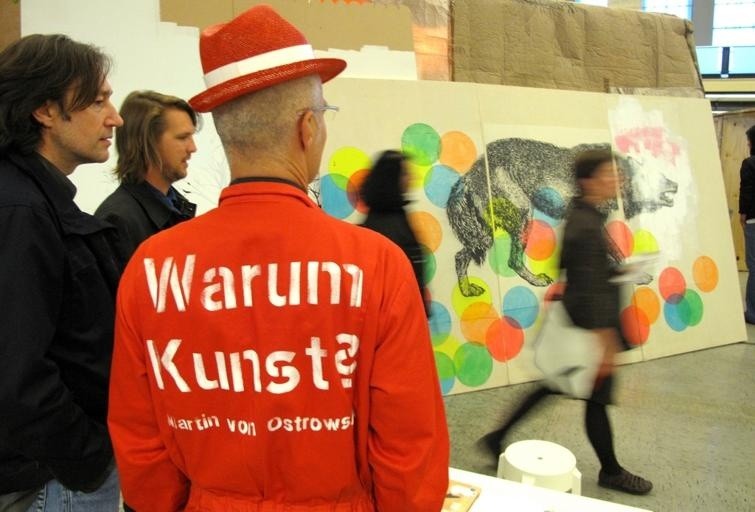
[[188, 6, 345, 114]]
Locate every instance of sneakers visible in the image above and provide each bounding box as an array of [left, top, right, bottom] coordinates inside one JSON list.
[[599, 466, 653, 495], [485, 431, 502, 460]]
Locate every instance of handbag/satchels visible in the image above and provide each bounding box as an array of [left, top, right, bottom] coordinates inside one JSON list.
[[533, 299, 602, 400]]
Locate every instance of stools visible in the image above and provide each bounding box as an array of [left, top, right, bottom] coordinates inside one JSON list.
[[496, 439, 582, 496]]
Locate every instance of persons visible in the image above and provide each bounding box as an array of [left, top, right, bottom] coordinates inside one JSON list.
[[0, 33, 128, 512], [472, 146, 654, 495], [350, 151, 435, 323], [737, 124, 755, 328], [105, 3, 451, 512], [90, 89, 201, 271]]
